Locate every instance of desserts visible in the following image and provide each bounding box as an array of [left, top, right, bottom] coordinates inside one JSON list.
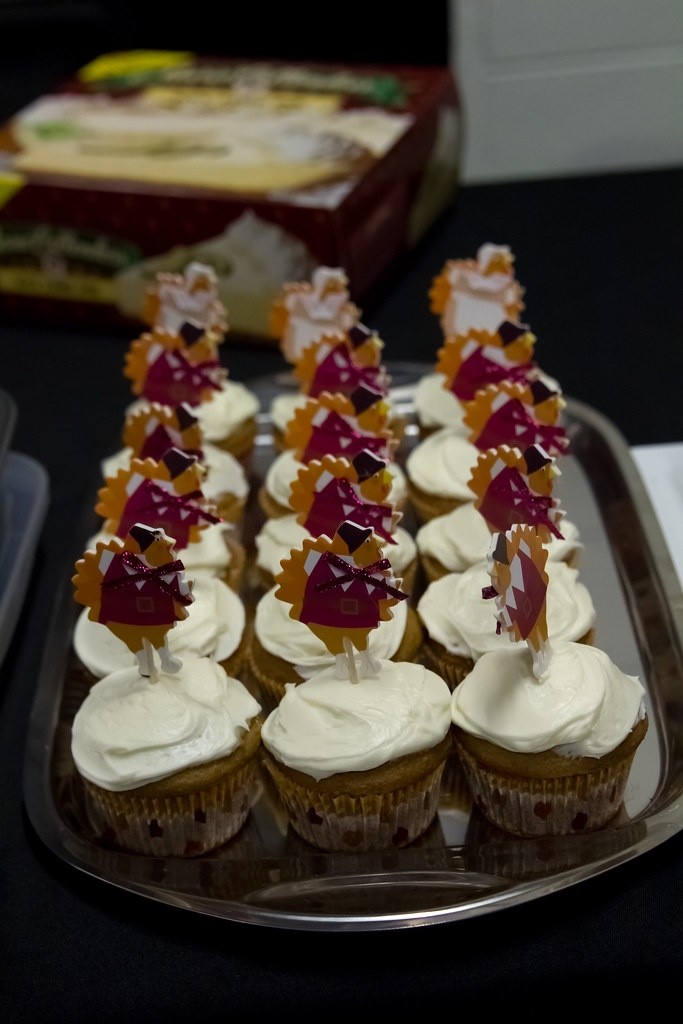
[[69, 240, 649, 861]]
